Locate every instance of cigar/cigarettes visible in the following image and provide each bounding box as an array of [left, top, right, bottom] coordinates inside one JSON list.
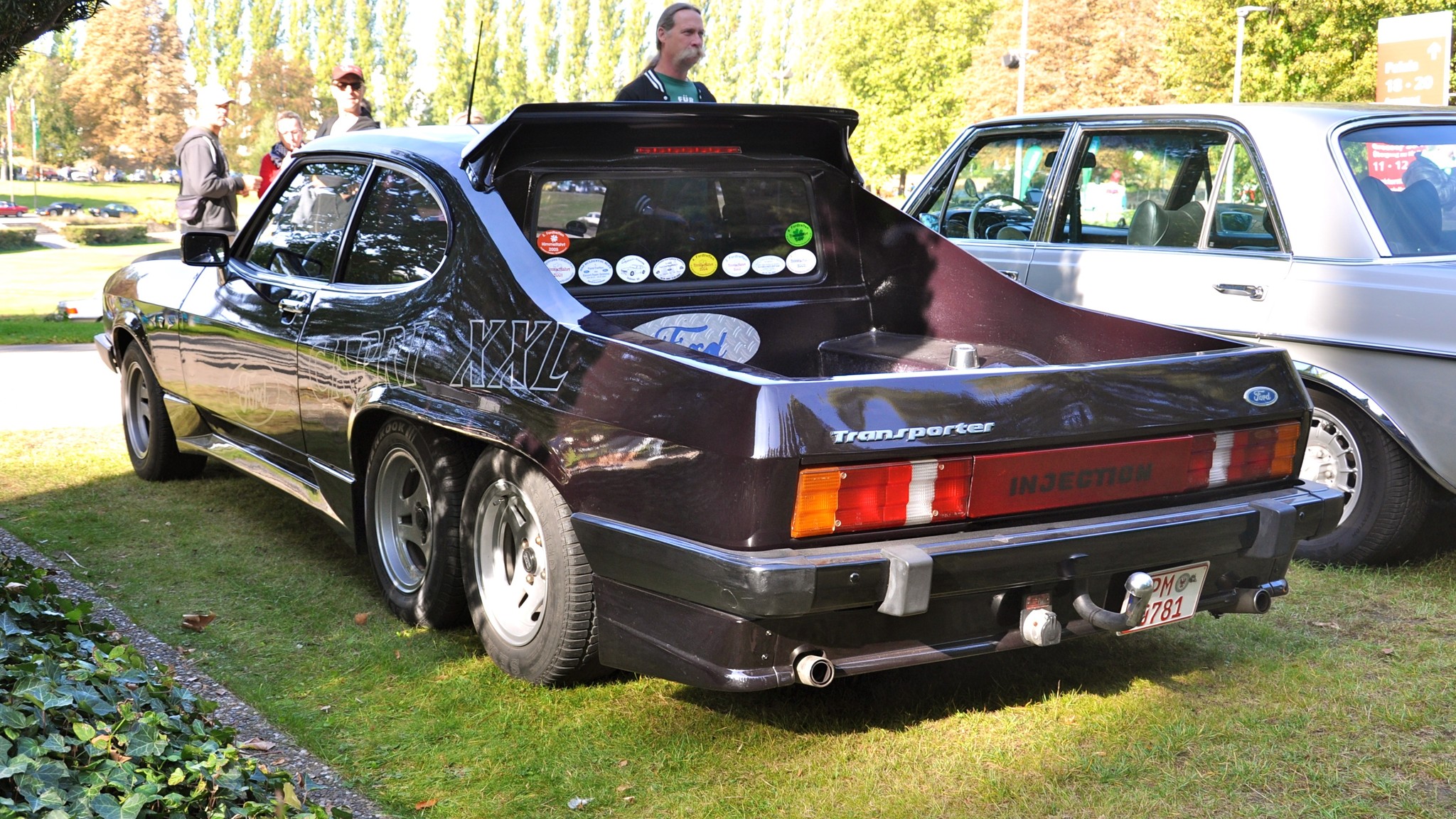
[[224, 117, 235, 125]]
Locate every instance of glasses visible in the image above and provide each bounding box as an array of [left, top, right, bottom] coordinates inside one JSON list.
[[331, 82, 362, 89]]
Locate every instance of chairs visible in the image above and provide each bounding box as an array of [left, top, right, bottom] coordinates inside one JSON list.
[[1233, 207, 1281, 252], [1360, 176, 1442, 253], [1126, 199, 1206, 248]]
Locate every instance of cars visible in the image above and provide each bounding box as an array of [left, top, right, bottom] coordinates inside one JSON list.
[[894, 100, 1456, 572], [37, 201, 82, 217], [0, 201, 29, 218], [0, 162, 182, 184], [89, 203, 138, 219]]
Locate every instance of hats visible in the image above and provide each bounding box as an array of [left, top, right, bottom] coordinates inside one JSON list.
[[330, 65, 364, 81], [197, 84, 237, 107]]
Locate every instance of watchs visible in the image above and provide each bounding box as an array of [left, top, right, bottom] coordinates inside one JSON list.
[[642, 203, 656, 215]]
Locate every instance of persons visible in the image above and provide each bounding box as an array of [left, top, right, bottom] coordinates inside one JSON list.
[[308, 65, 380, 235], [1402, 144, 1456, 223], [67, 166, 74, 181], [88, 165, 98, 182], [176, 83, 262, 245], [258, 111, 306, 203], [112, 173, 118, 181], [597, 3, 718, 239]]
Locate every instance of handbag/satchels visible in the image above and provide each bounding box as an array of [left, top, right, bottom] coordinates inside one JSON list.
[[176, 195, 203, 220]]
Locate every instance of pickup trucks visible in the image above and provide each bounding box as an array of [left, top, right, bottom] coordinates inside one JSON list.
[[94, 98, 1355, 691]]
